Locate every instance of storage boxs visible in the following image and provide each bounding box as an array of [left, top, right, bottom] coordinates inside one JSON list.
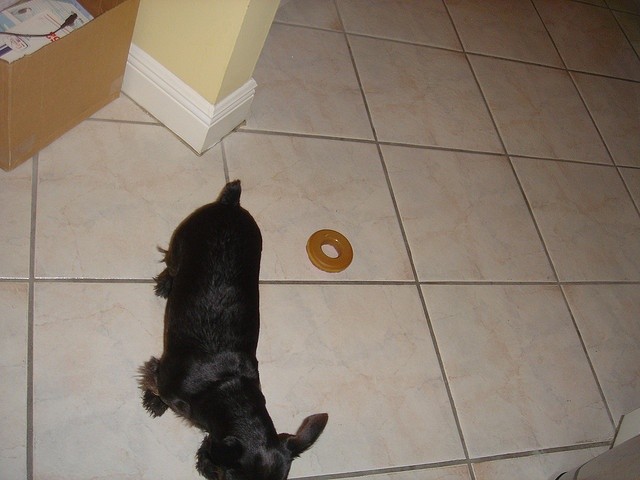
[[1, 2, 142, 171]]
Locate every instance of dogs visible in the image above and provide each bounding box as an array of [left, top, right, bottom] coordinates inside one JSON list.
[[132, 179, 329, 476]]
